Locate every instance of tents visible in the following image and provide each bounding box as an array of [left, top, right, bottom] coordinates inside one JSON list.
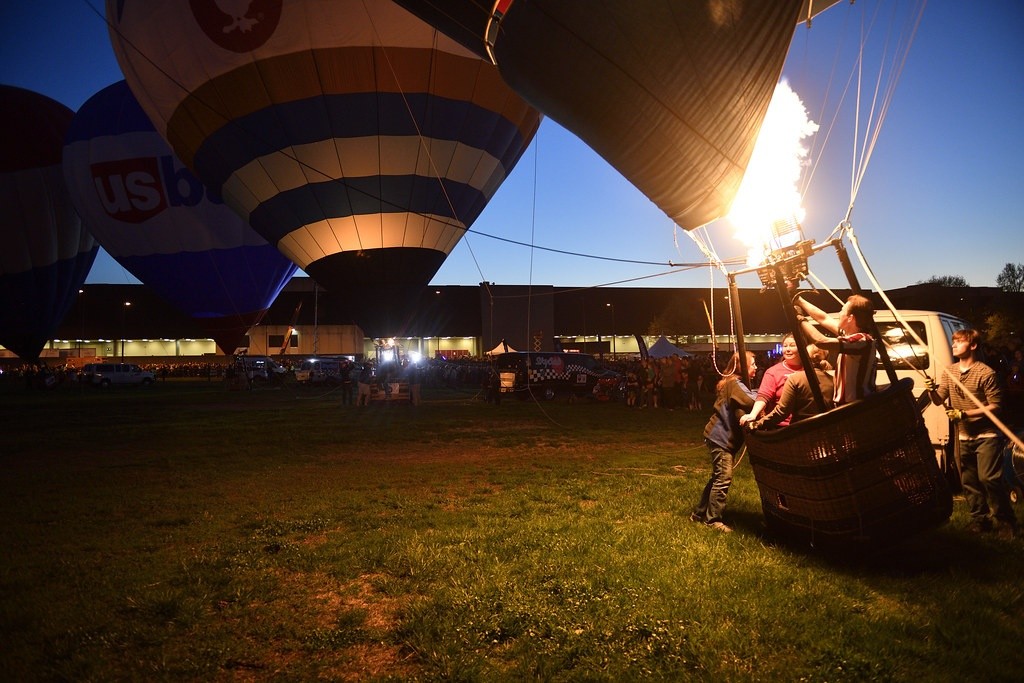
[[646, 336, 687, 358]]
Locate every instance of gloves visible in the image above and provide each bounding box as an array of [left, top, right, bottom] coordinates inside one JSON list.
[[925, 375, 936, 391], [945, 408, 962, 421]]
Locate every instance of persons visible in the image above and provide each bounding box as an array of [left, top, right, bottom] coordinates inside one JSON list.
[[794, 291, 876, 406], [928, 328, 1015, 534], [226, 364, 233, 385], [162, 367, 168, 382], [357, 365, 371, 407], [693, 348, 759, 532], [207, 364, 211, 382], [592, 353, 782, 415], [738, 333, 834, 429], [410, 368, 423, 406], [275, 360, 313, 376]]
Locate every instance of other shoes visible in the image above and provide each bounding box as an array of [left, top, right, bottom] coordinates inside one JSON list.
[[962, 517, 991, 536], [690, 513, 702, 522], [998, 520, 1014, 541], [705, 521, 730, 532]]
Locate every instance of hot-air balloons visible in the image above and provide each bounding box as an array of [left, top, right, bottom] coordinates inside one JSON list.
[[105, 1, 544, 402], [0, 84, 99, 391], [392, 0, 957, 563], [65, 80, 300, 394]]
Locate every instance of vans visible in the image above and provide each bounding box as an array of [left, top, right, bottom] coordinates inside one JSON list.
[[295, 359, 347, 386], [805, 310, 969, 496], [89, 363, 155, 389], [496, 352, 628, 402]]
